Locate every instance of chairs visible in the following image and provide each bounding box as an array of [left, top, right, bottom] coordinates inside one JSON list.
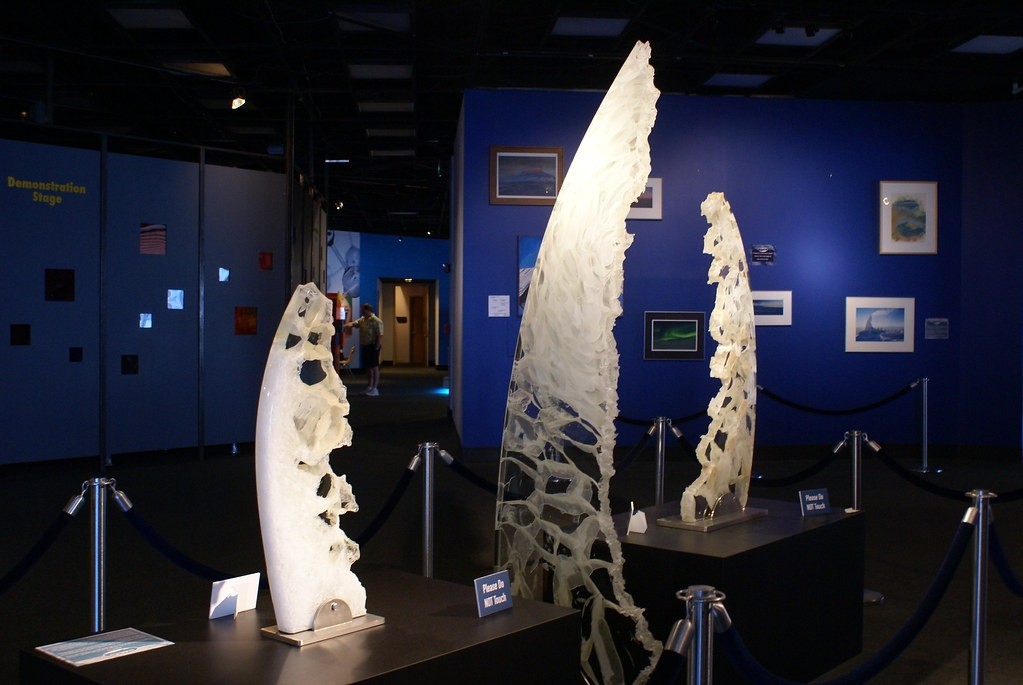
[[339, 346, 357, 379]]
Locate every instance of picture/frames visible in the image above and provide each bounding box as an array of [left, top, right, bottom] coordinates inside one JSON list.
[[627, 175, 663, 221], [752, 289, 793, 327], [643, 311, 706, 361], [880, 180, 938, 255], [489, 145, 564, 206], [845, 296, 915, 352], [517, 233, 541, 318]]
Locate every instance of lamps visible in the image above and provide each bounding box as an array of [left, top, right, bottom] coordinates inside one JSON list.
[[221, 83, 246, 110]]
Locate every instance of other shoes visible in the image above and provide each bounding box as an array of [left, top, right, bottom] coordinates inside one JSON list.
[[359, 387, 372, 396], [366, 388, 379, 396]]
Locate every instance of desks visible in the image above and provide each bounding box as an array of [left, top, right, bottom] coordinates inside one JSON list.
[[16, 565, 581, 685], [546, 495, 866, 685]]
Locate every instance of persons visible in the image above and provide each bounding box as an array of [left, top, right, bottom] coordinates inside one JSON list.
[[341, 302, 384, 397]]
